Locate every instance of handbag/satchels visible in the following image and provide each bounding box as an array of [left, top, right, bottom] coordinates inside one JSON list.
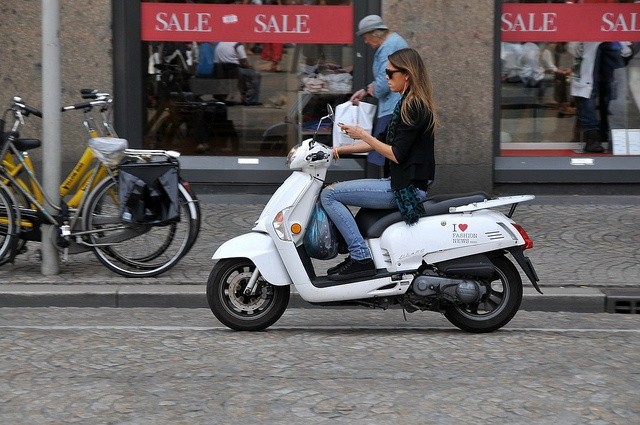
[[118, 162, 182, 227]]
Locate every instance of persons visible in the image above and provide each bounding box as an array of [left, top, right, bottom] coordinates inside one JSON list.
[[571, 41, 634, 153], [327, 47, 439, 283], [501, 42, 577, 118], [194, 41, 219, 78], [261, 42, 284, 72], [214, 41, 263, 106], [350, 14, 410, 179]]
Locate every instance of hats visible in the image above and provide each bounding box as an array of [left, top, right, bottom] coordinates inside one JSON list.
[[356, 15, 388, 37]]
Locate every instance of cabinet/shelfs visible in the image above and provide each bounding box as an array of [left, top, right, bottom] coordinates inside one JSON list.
[[295, 89, 354, 145]]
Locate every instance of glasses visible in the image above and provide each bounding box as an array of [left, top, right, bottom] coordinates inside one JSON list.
[[385, 68, 407, 78]]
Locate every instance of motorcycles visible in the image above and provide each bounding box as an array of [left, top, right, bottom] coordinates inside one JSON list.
[[207, 104, 544, 333], [261, 91, 313, 151]]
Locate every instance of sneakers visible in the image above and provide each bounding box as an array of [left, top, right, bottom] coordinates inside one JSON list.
[[327, 257, 375, 281], [327, 256, 351, 274]]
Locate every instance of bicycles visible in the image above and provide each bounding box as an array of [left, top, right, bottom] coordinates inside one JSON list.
[[0, 88, 202, 278], [145, 63, 238, 155]]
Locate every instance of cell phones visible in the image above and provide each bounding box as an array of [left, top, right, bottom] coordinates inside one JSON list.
[[337, 122, 344, 126]]
